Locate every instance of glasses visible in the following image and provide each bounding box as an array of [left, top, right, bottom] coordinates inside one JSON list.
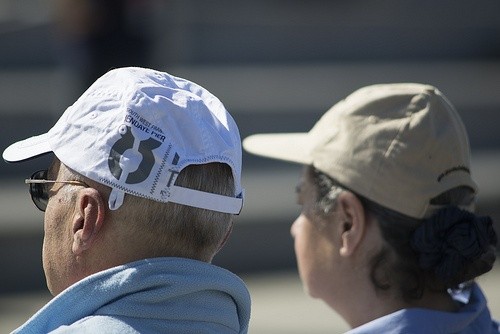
[[24, 169, 94, 211]]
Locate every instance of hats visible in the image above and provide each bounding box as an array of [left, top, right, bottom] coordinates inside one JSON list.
[[2, 66, 247, 216], [242, 82, 480, 219]]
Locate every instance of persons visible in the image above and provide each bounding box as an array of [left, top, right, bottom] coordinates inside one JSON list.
[[242, 82, 500, 334], [0, 67, 252, 334]]
